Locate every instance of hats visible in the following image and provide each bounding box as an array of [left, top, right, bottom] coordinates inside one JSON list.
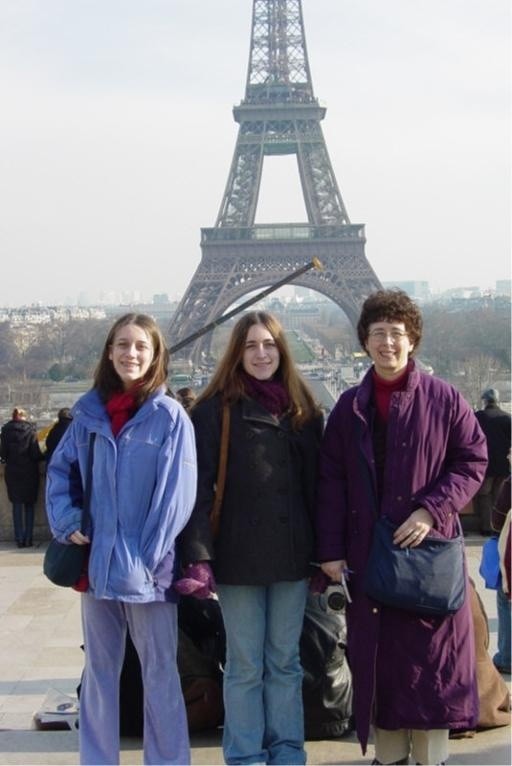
[[481, 388, 498, 400]]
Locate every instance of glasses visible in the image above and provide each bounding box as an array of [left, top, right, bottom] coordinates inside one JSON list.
[[368, 328, 411, 342]]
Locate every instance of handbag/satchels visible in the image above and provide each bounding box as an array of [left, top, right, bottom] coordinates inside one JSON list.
[[43, 537, 86, 587], [366, 516, 466, 613]]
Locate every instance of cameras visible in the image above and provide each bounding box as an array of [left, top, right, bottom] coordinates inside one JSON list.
[[326, 586, 347, 616]]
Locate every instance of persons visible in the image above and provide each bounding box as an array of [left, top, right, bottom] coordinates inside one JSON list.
[[488, 474, 512, 674], [175, 311, 328, 765], [467, 388, 512, 538], [0, 407, 46, 549], [315, 284, 489, 765], [47, 407, 73, 464], [176, 389, 197, 414], [46, 313, 199, 766]]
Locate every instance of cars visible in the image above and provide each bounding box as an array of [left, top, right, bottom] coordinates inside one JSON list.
[[169, 374, 198, 390], [32, 415, 56, 441]]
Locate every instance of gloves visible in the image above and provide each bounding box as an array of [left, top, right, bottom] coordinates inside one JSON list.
[[172, 560, 216, 599]]
